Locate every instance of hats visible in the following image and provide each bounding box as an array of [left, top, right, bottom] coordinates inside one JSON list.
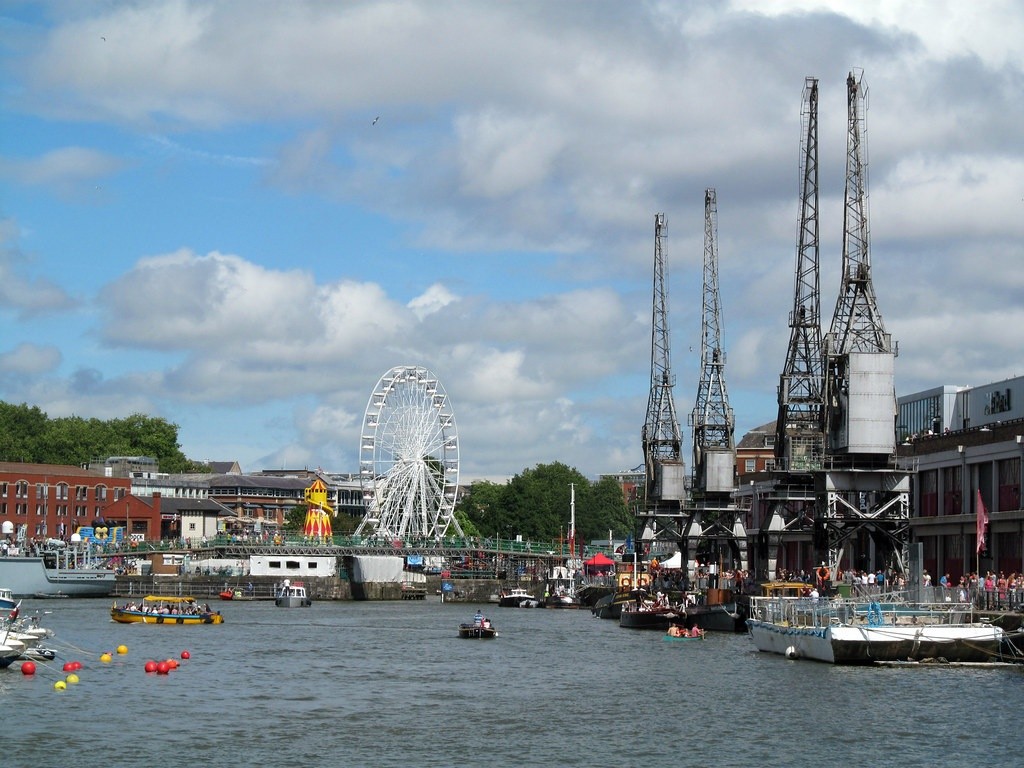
[[198, 606, 201, 608], [132, 602, 134, 604], [478, 610, 481, 612]]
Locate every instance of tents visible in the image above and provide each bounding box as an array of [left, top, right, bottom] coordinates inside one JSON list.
[[658, 552, 696, 571], [583, 551, 618, 581]]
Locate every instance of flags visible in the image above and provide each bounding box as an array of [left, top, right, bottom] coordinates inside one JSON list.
[[977, 489, 988, 553]]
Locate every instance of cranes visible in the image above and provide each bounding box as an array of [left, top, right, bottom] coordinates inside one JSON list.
[[758, 73, 921, 596], [627, 212, 692, 569], [677, 185, 755, 593]]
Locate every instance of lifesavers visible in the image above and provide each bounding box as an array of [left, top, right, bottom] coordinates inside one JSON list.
[[638, 577, 648, 586], [622, 577, 631, 587], [156, 617, 164, 623], [817, 568, 831, 580], [177, 617, 184, 624]]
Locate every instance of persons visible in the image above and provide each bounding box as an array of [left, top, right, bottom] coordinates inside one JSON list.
[[558, 582, 566, 597], [284, 577, 291, 597], [1, 530, 494, 575], [597, 571, 604, 586], [246, 581, 254, 598], [526, 540, 531, 553], [126, 601, 201, 615], [475, 609, 492, 629], [652, 568, 1024, 613]]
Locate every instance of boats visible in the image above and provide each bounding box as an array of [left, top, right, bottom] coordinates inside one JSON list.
[[499, 588, 535, 608], [619, 553, 746, 632], [518, 600, 539, 608], [34, 590, 69, 600], [274, 584, 310, 608], [0, 587, 19, 611], [458, 618, 497, 639], [661, 631, 706, 643], [0, 598, 59, 669], [0, 543, 117, 599], [591, 550, 658, 620], [744, 583, 1005, 666], [110, 595, 225, 624]]
